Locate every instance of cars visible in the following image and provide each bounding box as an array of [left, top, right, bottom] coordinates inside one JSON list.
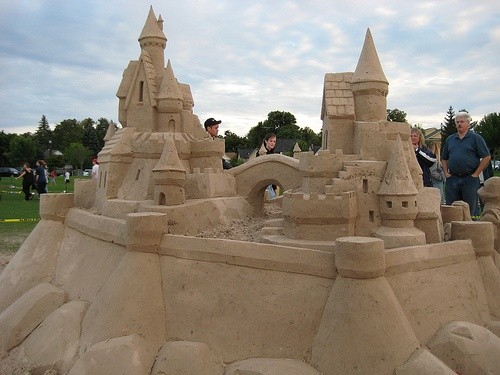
[[491, 160, 500, 170], [83, 169, 92, 176], [57, 168, 72, 176]]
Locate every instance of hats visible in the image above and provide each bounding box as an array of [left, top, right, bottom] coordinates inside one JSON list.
[[204, 118, 221, 127], [92, 159, 97, 163]]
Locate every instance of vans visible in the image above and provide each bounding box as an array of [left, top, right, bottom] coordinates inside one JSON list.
[[0, 167, 19, 178]]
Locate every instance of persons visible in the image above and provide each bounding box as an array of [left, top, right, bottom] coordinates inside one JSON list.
[[16, 160, 56, 201], [411, 128, 437, 187], [256, 134, 277, 190], [431, 112, 494, 221], [204, 118, 233, 170], [91, 159, 99, 180]]
